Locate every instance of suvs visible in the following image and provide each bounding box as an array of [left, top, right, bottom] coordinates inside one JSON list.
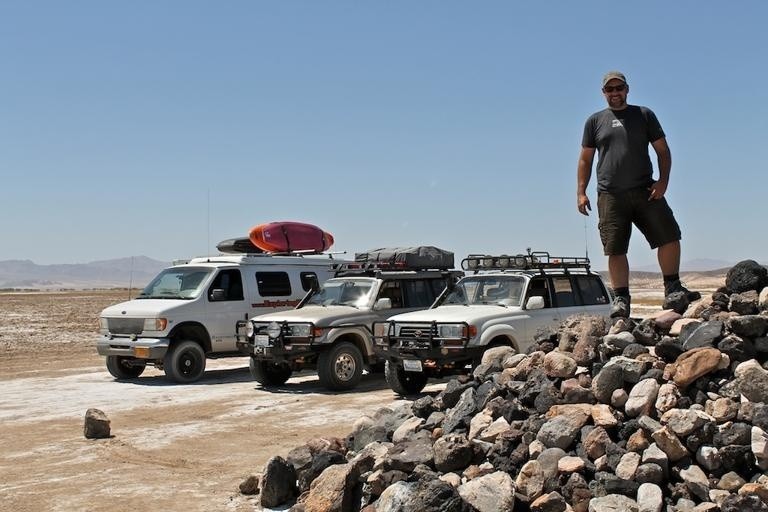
[[376, 252, 624, 395], [237, 248, 467, 396], [98, 252, 348, 388]]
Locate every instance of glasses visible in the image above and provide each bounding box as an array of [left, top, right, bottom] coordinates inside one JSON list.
[[605, 83, 626, 93]]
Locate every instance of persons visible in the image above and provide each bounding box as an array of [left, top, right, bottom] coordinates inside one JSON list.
[[578, 72, 700, 318]]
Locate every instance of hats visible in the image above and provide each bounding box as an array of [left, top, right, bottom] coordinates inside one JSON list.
[[600, 70, 626, 90]]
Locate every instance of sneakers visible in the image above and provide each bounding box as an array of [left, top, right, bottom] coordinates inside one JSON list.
[[610, 295, 630, 318], [662, 286, 701, 302]]
